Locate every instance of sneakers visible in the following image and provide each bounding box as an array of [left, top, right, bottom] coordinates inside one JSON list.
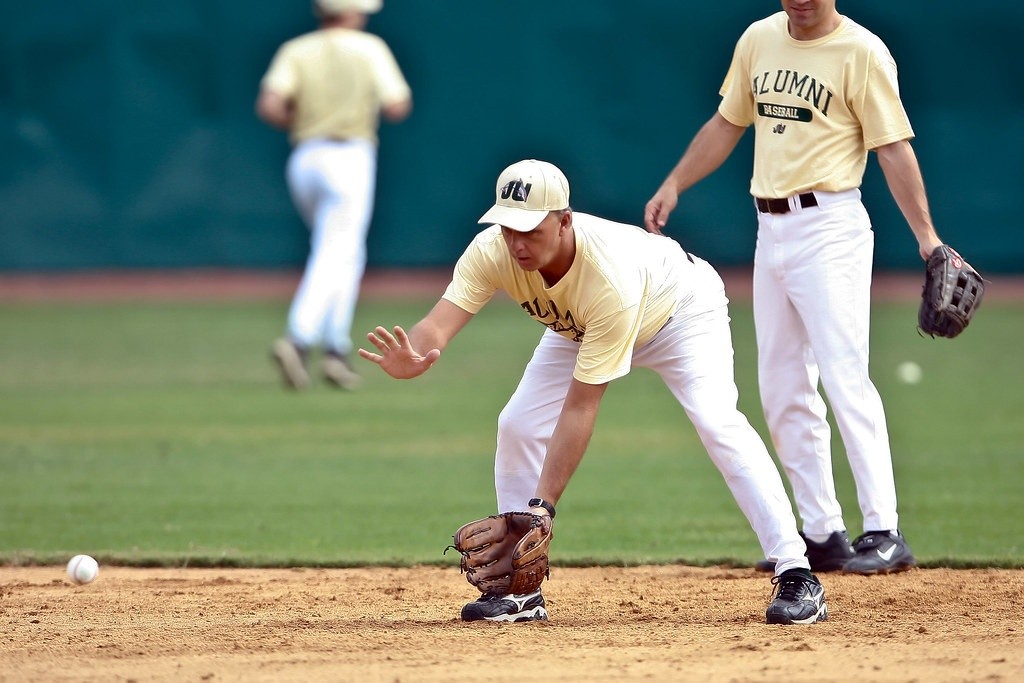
[[843, 530, 914, 575], [756, 530, 856, 572], [461, 586, 549, 623], [272, 339, 309, 388], [321, 351, 357, 389], [765, 568, 827, 624]]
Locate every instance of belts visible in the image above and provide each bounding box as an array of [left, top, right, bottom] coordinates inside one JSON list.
[[756, 193, 817, 214], [684, 249, 693, 265]]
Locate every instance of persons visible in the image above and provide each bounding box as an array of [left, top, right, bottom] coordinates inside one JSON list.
[[253, 0, 413, 392], [644, 0, 946, 572], [356, 158, 827, 628]]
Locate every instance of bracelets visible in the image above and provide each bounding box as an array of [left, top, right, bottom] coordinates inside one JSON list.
[[528, 498, 556, 520]]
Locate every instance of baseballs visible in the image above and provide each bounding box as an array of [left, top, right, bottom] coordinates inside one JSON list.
[[66, 554, 100, 586]]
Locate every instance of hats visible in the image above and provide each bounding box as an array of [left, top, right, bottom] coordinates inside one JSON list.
[[317, 0, 382, 15], [476, 159, 569, 233]]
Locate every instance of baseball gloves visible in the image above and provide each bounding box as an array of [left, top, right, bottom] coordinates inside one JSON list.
[[440, 511, 555, 596], [915, 242, 993, 340]]
